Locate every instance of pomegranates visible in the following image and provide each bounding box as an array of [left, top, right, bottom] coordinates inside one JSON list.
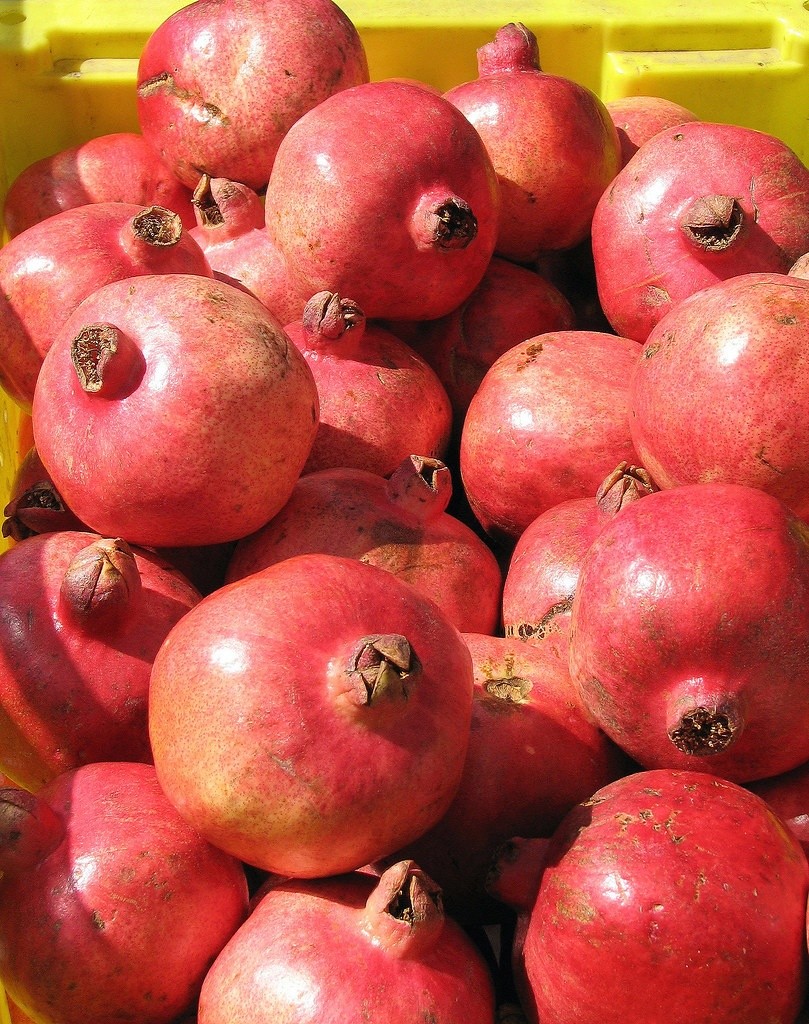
[[0, 0, 809, 1024]]
